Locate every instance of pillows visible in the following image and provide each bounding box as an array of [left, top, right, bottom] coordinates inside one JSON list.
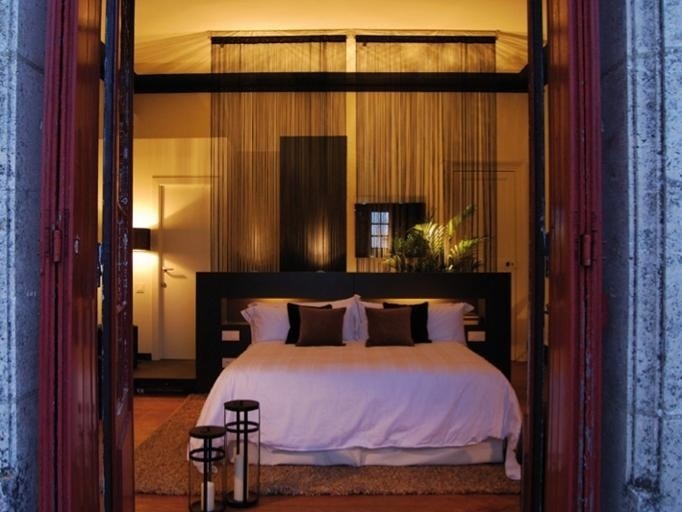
[[240, 294, 475, 347]]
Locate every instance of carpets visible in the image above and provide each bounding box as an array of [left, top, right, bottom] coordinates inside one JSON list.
[[134, 392, 523, 495]]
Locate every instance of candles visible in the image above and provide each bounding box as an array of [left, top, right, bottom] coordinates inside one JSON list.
[[200, 481, 215, 511], [233, 454, 249, 502]]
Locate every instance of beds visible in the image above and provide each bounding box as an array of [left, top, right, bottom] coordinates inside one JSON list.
[[187, 271, 523, 482]]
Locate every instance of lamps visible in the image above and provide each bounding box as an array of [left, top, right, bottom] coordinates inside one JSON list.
[[131, 227, 151, 252]]
